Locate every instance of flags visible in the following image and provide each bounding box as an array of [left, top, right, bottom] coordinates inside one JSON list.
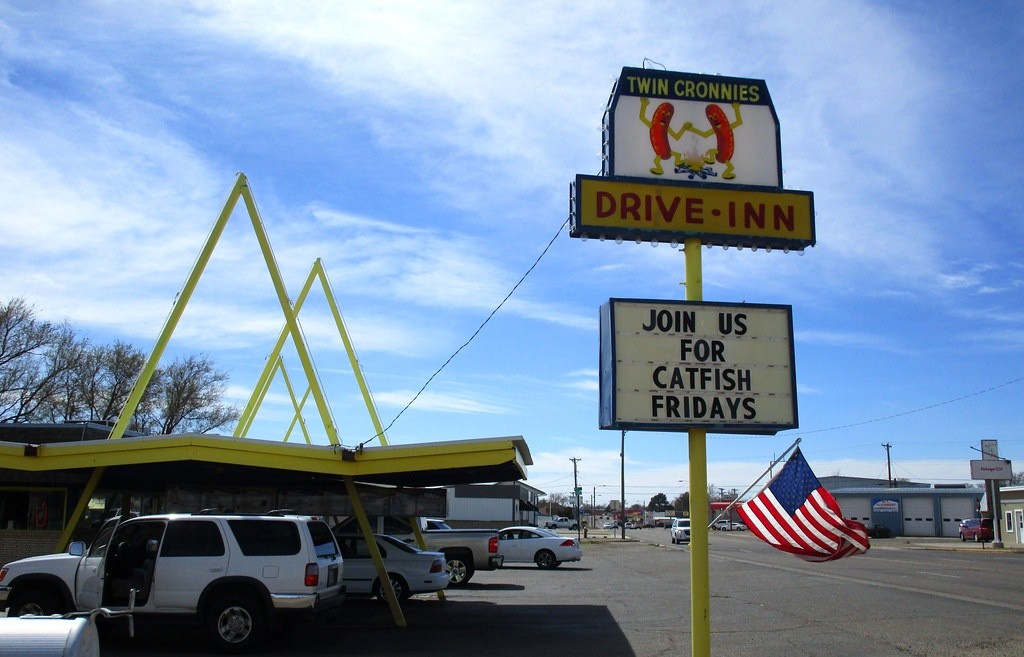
[[731, 442, 872, 563]]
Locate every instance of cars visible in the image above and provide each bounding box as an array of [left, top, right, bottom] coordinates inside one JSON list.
[[625, 522, 631, 528], [498, 526, 583, 570], [712, 520, 731, 530], [546, 517, 577, 531], [721, 523, 749, 531], [425, 518, 452, 530], [670, 518, 691, 544], [603, 523, 612, 530], [959, 517, 994, 541], [333, 533, 449, 608]]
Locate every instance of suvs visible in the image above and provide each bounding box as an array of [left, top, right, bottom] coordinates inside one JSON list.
[[0, 508, 350, 653]]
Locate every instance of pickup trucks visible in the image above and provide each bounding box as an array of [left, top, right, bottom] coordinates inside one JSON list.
[[329, 513, 505, 588]]
